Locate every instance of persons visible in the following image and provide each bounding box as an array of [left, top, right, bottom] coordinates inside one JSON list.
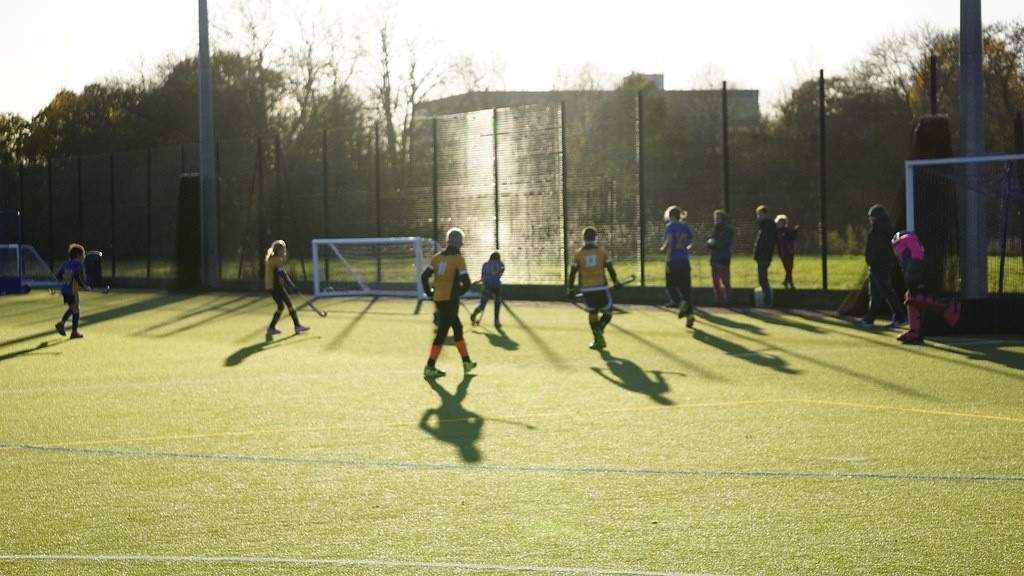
[[567, 226, 623, 349], [852, 204, 909, 329], [470, 252, 505, 326], [888, 227, 961, 343], [421, 228, 476, 377], [659, 205, 695, 327], [55, 244, 91, 340], [706, 210, 734, 308], [266, 239, 311, 334], [772, 215, 799, 289], [752, 205, 776, 306]]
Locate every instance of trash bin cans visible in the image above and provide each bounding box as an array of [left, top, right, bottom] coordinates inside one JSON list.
[[84, 251, 102, 288]]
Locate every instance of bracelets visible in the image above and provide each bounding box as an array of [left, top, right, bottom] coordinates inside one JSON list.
[[568, 288, 575, 291]]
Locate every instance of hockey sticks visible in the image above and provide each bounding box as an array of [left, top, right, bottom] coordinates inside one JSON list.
[[470, 279, 483, 286], [80, 285, 110, 294], [297, 291, 328, 317], [575, 274, 636, 298]]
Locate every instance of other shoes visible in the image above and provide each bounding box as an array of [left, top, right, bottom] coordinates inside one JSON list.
[[471, 314, 476, 321], [678, 302, 689, 318], [71, 333, 84, 339], [886, 321, 907, 331], [464, 362, 476, 372], [424, 366, 446, 376], [56, 323, 67, 335], [897, 329, 923, 343], [295, 325, 310, 334], [686, 316, 695, 328], [590, 340, 606, 350], [853, 319, 873, 330], [268, 328, 281, 334], [945, 302, 961, 326], [495, 322, 502, 327]]
